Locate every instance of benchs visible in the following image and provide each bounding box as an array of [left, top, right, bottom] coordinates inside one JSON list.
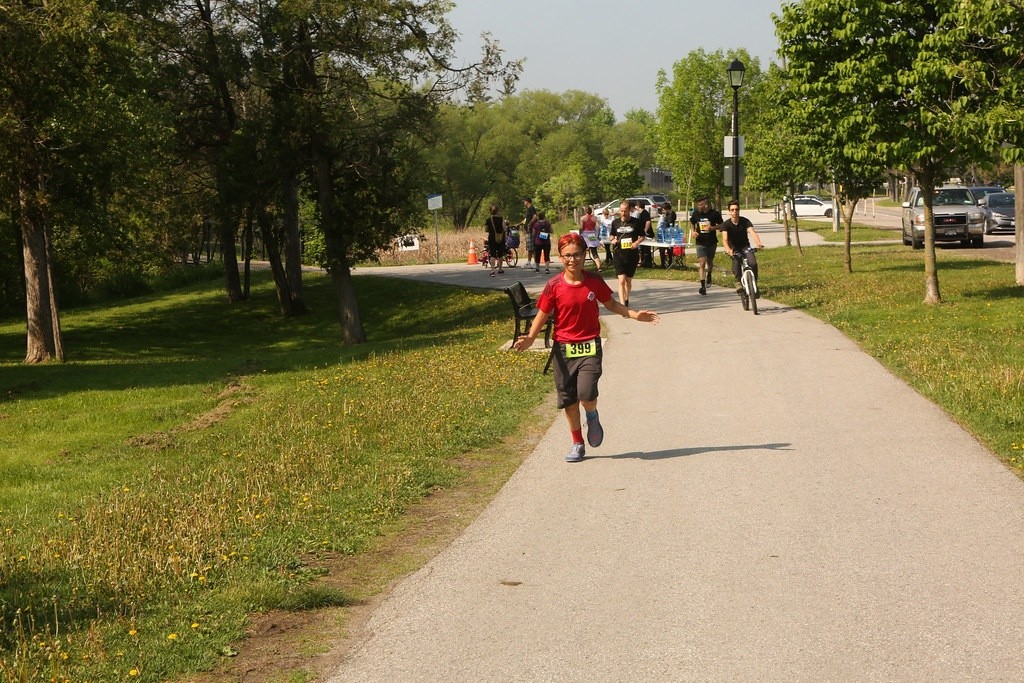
[[503, 281, 553, 351]]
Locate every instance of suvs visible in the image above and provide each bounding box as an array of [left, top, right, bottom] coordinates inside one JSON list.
[[901, 185, 985, 249]]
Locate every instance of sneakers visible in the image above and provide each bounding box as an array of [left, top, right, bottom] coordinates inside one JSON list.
[[565, 441, 586, 461], [586, 410, 603, 447]]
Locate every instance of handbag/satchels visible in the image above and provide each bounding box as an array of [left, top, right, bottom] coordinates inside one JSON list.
[[673, 236, 686, 256], [495, 233, 503, 243]]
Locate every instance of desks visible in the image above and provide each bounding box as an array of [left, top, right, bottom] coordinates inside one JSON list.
[[586, 240, 612, 269], [639, 243, 693, 273]]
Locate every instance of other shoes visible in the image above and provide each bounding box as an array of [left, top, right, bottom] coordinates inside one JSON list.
[[497, 270, 505, 275], [535, 269, 540, 273], [625, 299, 628, 306], [755, 287, 761, 299], [699, 288, 707, 295], [490, 272, 495, 277], [706, 275, 711, 288], [545, 269, 551, 274], [521, 263, 532, 268], [597, 268, 603, 272], [736, 282, 744, 294]]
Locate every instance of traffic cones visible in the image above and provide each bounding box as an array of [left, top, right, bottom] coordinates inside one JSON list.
[[466, 238, 481, 265]]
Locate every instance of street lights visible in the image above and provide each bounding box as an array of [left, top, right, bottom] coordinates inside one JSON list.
[[725, 57, 747, 208]]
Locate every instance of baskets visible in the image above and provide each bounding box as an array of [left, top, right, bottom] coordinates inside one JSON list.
[[506, 235, 519, 248]]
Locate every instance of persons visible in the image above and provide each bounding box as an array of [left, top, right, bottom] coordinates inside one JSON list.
[[610, 201, 646, 307], [533, 212, 554, 273], [719, 200, 762, 300], [513, 197, 536, 268], [628, 202, 655, 267], [658, 202, 676, 269], [690, 198, 723, 295], [515, 233, 659, 462], [598, 208, 612, 263], [484, 205, 506, 277], [579, 206, 602, 272]]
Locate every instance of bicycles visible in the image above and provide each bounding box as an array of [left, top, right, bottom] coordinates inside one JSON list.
[[722, 245, 764, 314], [481, 223, 523, 268]]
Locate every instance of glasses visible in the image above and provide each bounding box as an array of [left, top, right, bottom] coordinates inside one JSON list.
[[730, 208, 739, 212], [560, 250, 584, 261]]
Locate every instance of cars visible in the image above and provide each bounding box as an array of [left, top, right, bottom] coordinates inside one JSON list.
[[784, 194, 824, 203], [980, 192, 1015, 235], [969, 186, 1007, 206], [633, 195, 672, 210], [592, 197, 660, 224], [784, 198, 835, 218]]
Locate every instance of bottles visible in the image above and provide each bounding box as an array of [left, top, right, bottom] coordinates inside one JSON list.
[[599, 223, 606, 237], [657, 223, 682, 244]]
